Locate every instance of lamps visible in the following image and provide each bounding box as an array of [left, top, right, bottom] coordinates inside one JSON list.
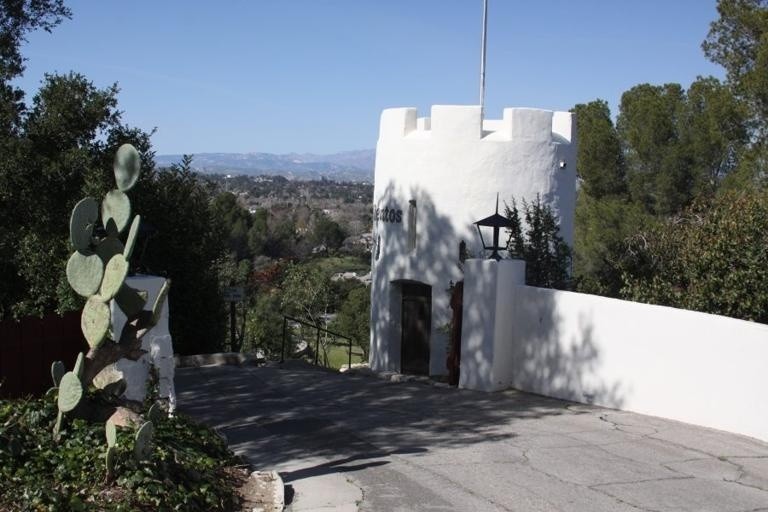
[[472, 191, 517, 261]]
